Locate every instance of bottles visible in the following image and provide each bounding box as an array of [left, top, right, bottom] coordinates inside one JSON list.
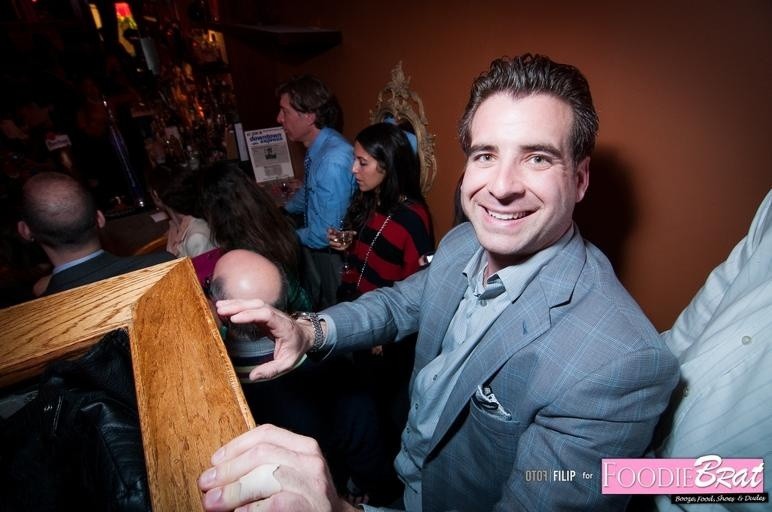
[[153, 69, 234, 163]]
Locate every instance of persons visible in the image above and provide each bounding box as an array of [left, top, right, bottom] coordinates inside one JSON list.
[[326, 121, 436, 364], [276, 74, 356, 309], [645, 185, 772, 511], [148, 162, 317, 313], [203, 248, 371, 508], [15, 169, 178, 298], [197, 51, 686, 512]]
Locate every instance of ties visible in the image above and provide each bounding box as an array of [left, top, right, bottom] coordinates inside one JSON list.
[[303, 151, 311, 228]]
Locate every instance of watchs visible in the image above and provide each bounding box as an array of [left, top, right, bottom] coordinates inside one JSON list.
[[291, 308, 324, 355]]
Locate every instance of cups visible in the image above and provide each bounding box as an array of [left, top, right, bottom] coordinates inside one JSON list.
[[330, 221, 353, 249]]
